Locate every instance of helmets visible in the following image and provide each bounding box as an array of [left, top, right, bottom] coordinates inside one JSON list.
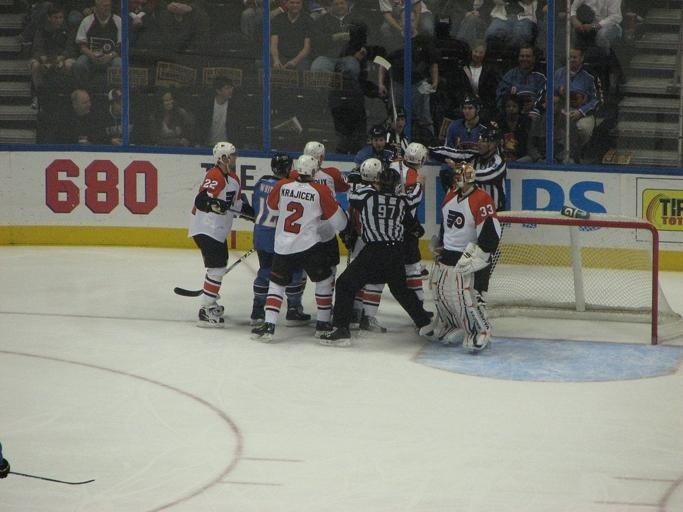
[[389, 105, 405, 121], [270, 152, 292, 176], [479, 129, 500, 144], [452, 161, 476, 193], [461, 93, 480, 116], [369, 124, 386, 138], [296, 154, 319, 176], [359, 158, 384, 182], [303, 141, 325, 163], [212, 141, 235, 165], [378, 168, 401, 193], [403, 143, 427, 169]]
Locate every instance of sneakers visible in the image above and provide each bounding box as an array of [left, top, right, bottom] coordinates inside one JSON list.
[[198, 305, 226, 324], [285, 302, 311, 322], [358, 315, 387, 334], [316, 321, 332, 332], [251, 322, 275, 339], [420, 300, 434, 318], [250, 299, 266, 321], [320, 325, 351, 341], [349, 309, 361, 326]]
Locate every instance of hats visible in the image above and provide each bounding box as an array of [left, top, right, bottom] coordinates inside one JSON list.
[[107, 88, 122, 101]]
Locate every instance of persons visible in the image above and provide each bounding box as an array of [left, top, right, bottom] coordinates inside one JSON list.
[[546, 45, 604, 149], [375, 9, 439, 137], [195, 76, 241, 148], [73, 1, 122, 73], [241, 0, 288, 41], [432, 164, 502, 350], [55, 90, 95, 144], [461, 40, 498, 109], [29, 6, 73, 108], [355, 128, 396, 166], [445, 97, 488, 150], [271, 0, 315, 70], [188, 141, 254, 328], [96, 87, 134, 145], [149, 91, 190, 147], [487, 0, 539, 56], [320, 168, 433, 346], [427, 130, 506, 293], [128, 0, 193, 67], [387, 110, 409, 157], [495, 45, 546, 118], [390, 142, 428, 307], [350, 158, 387, 335], [378, 0, 437, 34], [497, 93, 529, 136], [249, 154, 348, 342], [289, 141, 351, 296], [515, 92, 578, 164], [570, 0, 624, 68], [310, 0, 368, 78], [447, 0, 491, 54], [250, 152, 311, 327]]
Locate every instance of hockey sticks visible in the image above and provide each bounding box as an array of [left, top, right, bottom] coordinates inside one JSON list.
[[373, 56, 406, 195], [174, 247, 257, 297]]
